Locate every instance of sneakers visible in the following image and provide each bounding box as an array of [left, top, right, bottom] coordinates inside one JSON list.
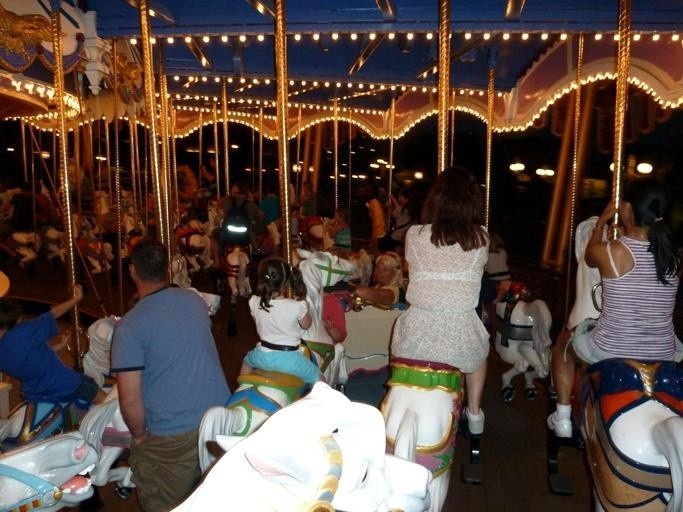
[[548, 413, 572, 438], [466, 406, 484, 435]]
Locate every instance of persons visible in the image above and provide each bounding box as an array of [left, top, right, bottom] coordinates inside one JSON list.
[[2, 153, 682, 440], [0, 283, 134, 501], [108, 236, 234, 512]]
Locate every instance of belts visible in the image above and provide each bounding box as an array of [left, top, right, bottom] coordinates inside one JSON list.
[[261, 342, 299, 351]]
